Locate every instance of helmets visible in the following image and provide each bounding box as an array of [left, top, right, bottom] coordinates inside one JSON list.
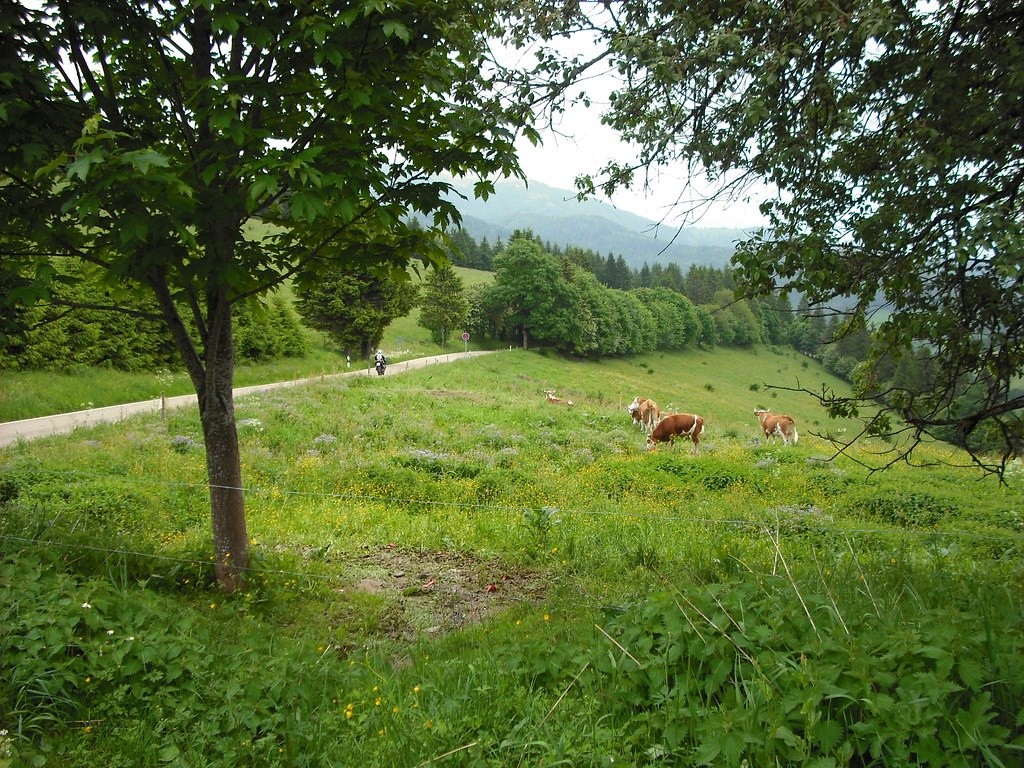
[[378, 350, 381, 353]]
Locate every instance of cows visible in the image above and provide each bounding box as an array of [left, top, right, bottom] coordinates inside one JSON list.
[[753, 407, 799, 449], [627, 396, 705, 455], [542, 389, 575, 409]]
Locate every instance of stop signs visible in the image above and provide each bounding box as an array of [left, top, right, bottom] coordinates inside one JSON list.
[[462, 333, 469, 341]]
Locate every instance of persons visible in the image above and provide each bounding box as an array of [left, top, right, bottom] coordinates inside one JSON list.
[[374, 350, 386, 372]]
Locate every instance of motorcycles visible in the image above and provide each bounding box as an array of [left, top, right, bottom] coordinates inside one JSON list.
[[373, 361, 387, 377]]
